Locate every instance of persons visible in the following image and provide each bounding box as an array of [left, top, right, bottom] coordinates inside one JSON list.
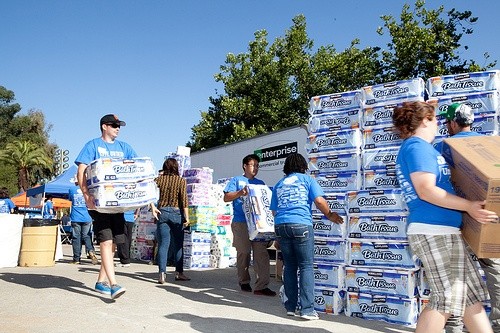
[[43, 194, 53, 218], [223, 154, 276, 296], [391, 101, 500, 333], [74, 114, 139, 299], [69, 182, 98, 265], [61, 214, 70, 225], [0, 187, 16, 214], [269, 153, 344, 319], [150, 158, 192, 284], [115, 208, 140, 267]]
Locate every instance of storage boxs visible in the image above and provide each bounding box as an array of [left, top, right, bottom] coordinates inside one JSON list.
[[440, 135, 500, 259]]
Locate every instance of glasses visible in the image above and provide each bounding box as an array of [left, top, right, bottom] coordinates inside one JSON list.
[[105, 123, 120, 129], [252, 164, 260, 168]]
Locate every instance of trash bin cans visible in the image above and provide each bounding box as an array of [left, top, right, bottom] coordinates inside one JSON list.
[[18, 218, 60, 267], [1, 213, 24, 268]]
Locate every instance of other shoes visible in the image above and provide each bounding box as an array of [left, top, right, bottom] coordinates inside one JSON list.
[[300, 310, 320, 320], [90, 251, 98, 265], [175, 273, 191, 281], [239, 281, 252, 292], [287, 309, 296, 316], [157, 272, 165, 284], [254, 287, 276, 296]]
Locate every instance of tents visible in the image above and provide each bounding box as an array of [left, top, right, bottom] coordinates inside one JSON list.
[[24, 164, 78, 218], [11, 184, 72, 218]]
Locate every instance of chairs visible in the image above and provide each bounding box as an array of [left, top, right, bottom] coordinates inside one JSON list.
[[60, 224, 72, 245]]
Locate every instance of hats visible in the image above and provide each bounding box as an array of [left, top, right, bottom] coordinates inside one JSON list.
[[100, 113, 126, 127], [439, 103, 474, 126]]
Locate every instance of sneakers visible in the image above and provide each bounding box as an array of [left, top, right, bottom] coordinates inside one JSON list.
[[111, 284, 126, 299], [95, 281, 111, 295]]
